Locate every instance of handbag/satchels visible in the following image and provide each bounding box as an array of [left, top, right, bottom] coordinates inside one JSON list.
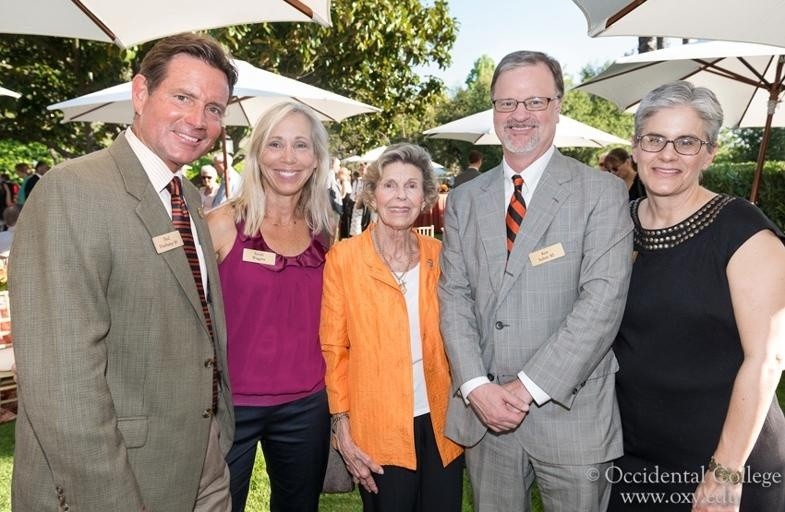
[[321, 427, 357, 496]]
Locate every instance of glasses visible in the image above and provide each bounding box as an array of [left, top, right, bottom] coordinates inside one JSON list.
[[637, 132, 713, 155], [491, 96, 559, 113]]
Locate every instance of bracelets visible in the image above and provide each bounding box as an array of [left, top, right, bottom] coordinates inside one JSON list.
[[709, 456, 745, 485], [331, 413, 349, 433]]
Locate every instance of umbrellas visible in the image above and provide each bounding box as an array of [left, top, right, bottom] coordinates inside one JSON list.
[[575, 0, 785, 48], [0, 0, 333, 51], [423, 108, 633, 148], [569, 41, 785, 204], [47, 59, 383, 200]]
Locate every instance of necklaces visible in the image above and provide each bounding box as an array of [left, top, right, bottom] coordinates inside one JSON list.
[[374, 228, 414, 294]]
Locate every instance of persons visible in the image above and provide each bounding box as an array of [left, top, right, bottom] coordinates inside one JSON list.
[[605, 148, 646, 201], [8, 32, 236, 512], [599, 153, 608, 170], [454, 151, 481, 189], [205, 103, 330, 512], [318, 142, 464, 512], [606, 80, 785, 512], [437, 50, 636, 512]]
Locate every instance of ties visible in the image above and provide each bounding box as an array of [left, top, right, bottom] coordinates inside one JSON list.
[[505, 174, 527, 261], [166, 176, 218, 415]]
